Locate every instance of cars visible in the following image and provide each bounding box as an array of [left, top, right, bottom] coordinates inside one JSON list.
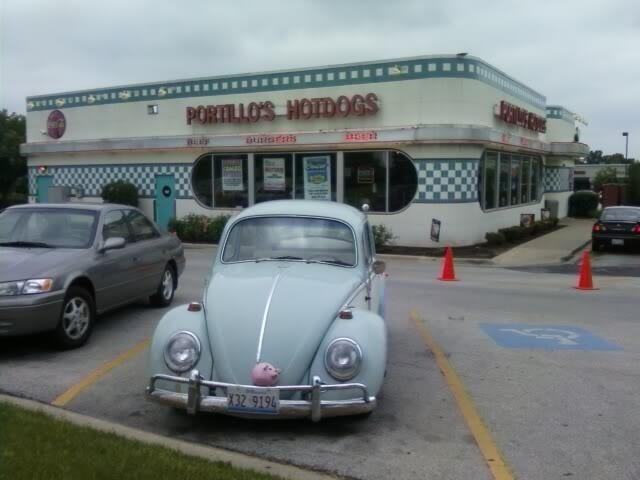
[[0, 203, 185, 349], [145, 199, 388, 421], [591, 206, 640, 251]]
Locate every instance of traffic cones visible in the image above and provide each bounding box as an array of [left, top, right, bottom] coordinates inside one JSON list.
[[574, 250, 598, 290], [440, 245, 459, 280]]
